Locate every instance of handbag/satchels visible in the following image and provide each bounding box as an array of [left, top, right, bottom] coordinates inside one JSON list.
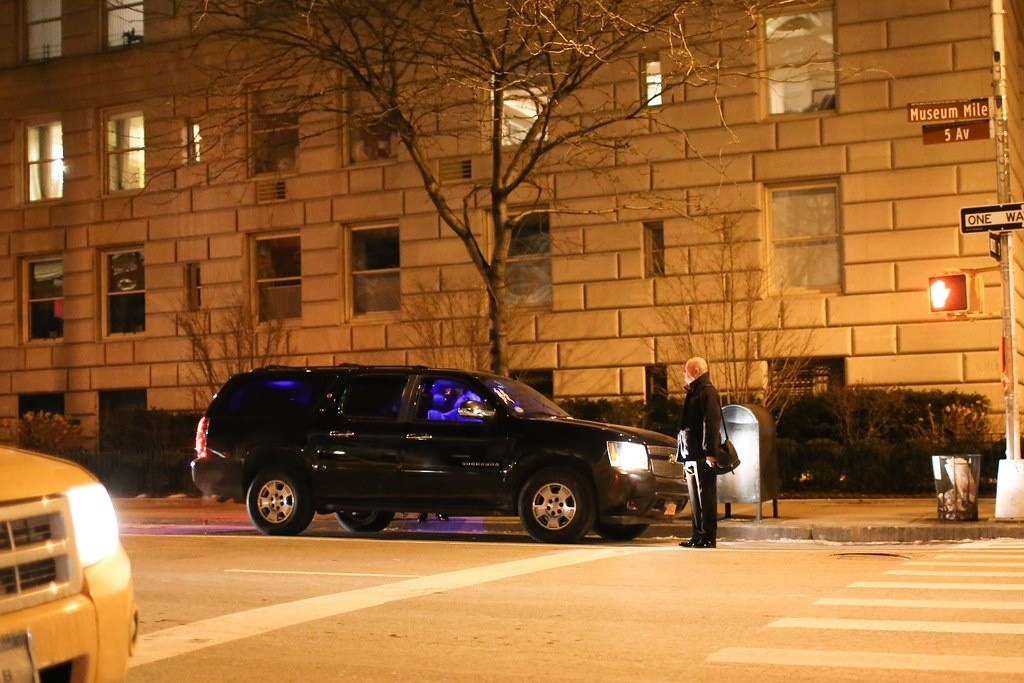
[[703, 440, 740, 476]]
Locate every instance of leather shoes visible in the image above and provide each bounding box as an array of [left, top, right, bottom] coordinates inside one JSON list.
[[679, 539, 716, 548]]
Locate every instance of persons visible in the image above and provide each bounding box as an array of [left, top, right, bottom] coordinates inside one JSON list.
[[417, 514, 450, 524], [674, 355, 722, 548]]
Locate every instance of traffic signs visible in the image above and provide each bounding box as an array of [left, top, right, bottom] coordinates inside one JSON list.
[[959, 202, 1024, 233]]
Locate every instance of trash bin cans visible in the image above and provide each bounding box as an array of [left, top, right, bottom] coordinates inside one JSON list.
[[930, 453, 983, 521], [714, 402, 779, 521]]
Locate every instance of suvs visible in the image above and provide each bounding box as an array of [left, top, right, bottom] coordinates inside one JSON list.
[[191, 362, 688, 544]]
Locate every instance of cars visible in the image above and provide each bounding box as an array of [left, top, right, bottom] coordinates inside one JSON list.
[[0, 442, 138, 683]]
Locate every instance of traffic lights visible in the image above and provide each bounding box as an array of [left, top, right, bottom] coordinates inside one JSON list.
[[928, 274, 970, 315]]
